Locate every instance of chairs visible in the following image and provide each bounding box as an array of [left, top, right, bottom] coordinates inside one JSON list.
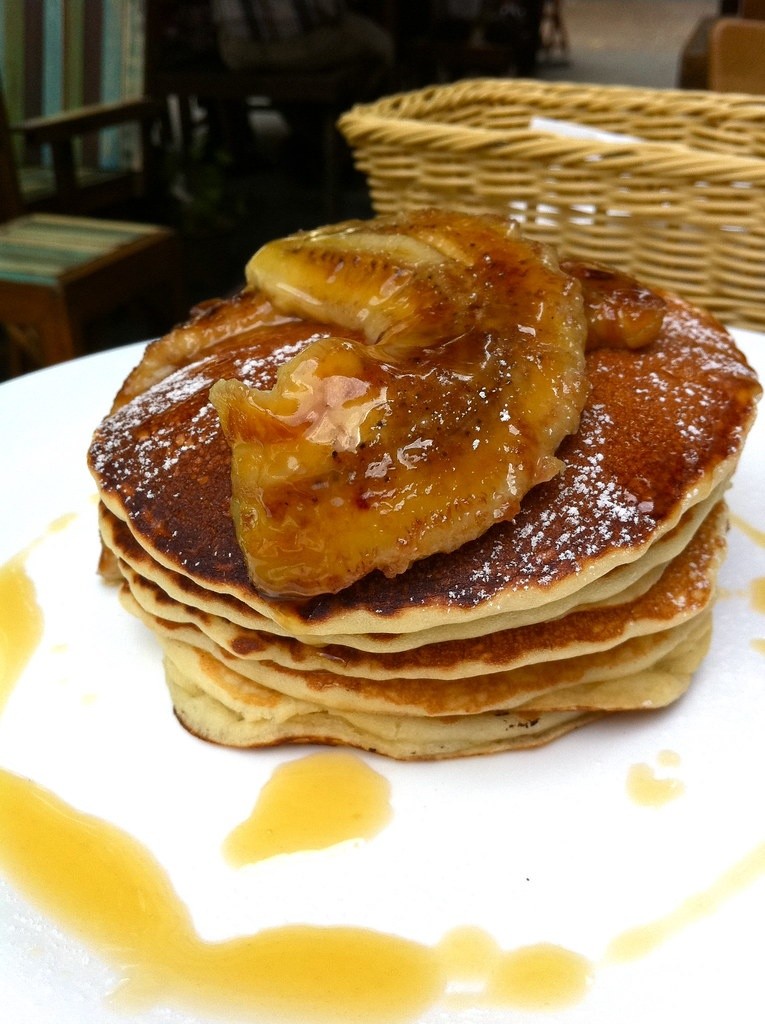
[[0, 88, 180, 364]]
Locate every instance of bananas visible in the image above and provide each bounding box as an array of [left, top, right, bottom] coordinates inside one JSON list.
[[206, 207, 591, 595], [117, 255, 673, 412]]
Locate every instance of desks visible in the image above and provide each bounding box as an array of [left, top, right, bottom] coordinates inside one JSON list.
[[0, 328, 765, 1024]]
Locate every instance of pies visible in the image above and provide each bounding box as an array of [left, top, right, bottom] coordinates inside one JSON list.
[[86, 275, 765, 762]]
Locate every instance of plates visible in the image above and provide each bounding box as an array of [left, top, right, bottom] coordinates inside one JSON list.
[[1, 325, 765, 1024]]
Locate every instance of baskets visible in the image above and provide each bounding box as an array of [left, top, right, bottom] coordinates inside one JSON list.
[[340, 76, 765, 332]]
